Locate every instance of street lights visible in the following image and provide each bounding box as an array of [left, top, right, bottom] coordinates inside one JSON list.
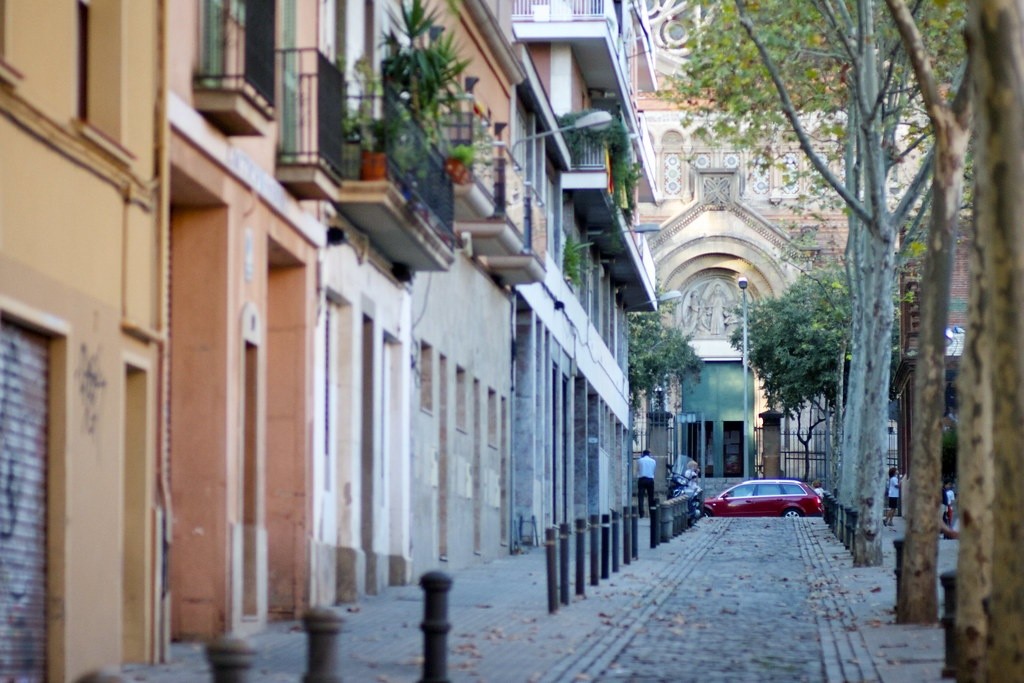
[[737, 275, 761, 480]]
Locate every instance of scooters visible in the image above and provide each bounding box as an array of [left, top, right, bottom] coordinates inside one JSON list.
[[663, 464, 705, 523]]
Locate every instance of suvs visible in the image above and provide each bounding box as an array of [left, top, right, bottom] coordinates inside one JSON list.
[[701, 478, 823, 520]]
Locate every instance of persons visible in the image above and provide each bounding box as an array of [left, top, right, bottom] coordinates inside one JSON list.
[[883, 465, 906, 527], [942, 479, 960, 540], [684, 460, 702, 492], [813, 479, 824, 500], [635, 451, 658, 519]]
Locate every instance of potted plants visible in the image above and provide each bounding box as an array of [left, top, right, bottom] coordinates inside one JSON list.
[[337, 1, 482, 258]]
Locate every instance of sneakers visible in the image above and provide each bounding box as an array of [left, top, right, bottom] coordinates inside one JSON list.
[[882, 517, 887, 526], [887, 520, 894, 526]]
